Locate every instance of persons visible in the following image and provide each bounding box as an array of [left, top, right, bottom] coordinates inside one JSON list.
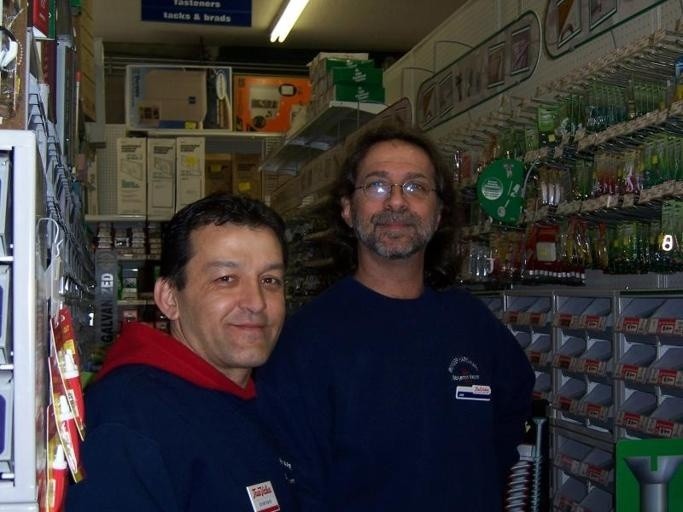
[[54, 190, 331, 509], [239, 129, 537, 509]]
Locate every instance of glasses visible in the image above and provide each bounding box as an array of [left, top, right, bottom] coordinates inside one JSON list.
[[349, 177, 440, 203]]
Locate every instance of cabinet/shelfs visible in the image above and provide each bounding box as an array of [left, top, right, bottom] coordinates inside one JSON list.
[[82, 52, 386, 378]]
